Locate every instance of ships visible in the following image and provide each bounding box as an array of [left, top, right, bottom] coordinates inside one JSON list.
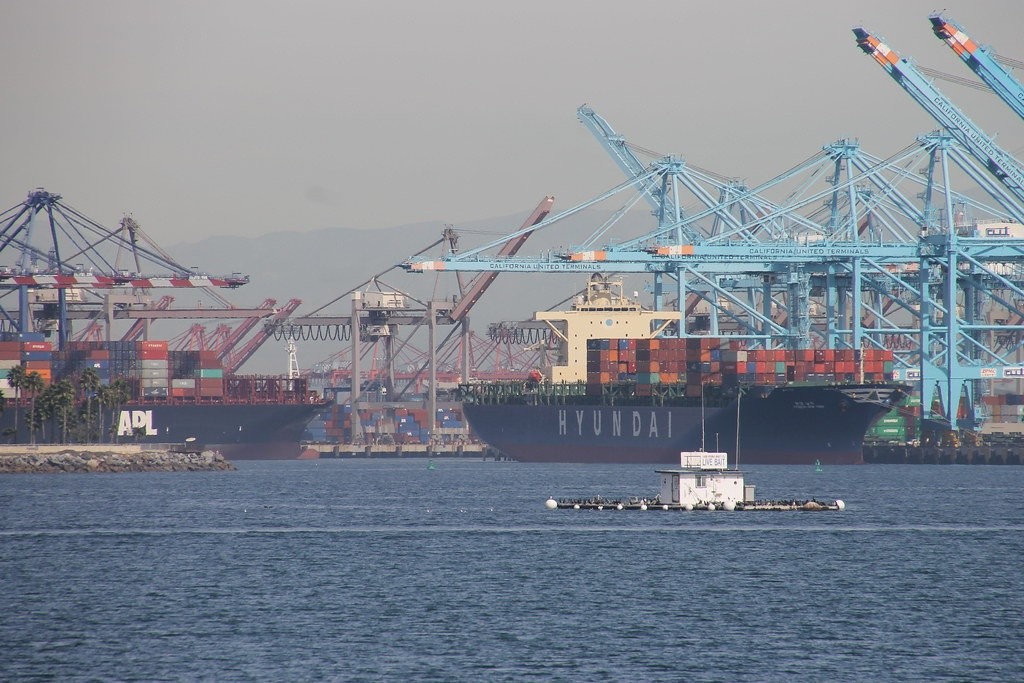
[[0, 396, 334, 461], [457, 278, 911, 461]]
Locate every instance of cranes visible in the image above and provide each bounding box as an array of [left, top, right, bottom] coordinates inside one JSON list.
[[0, 2, 1024, 435]]
